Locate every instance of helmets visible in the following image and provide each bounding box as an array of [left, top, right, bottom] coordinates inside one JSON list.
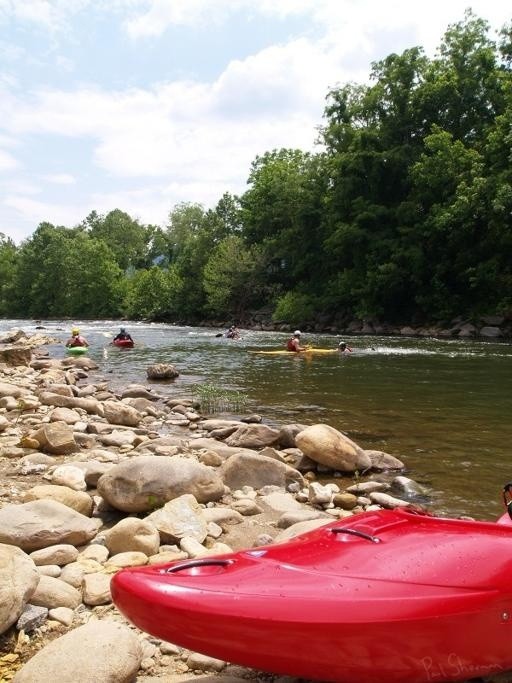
[[294, 330, 301, 336], [229, 326, 235, 331], [339, 342, 346, 346], [120, 327, 126, 332], [72, 327, 80, 336]]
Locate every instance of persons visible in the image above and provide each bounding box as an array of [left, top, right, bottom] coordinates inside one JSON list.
[[288, 330, 308, 352], [111, 327, 132, 341], [65, 329, 89, 348], [226, 324, 240, 339], [338, 341, 353, 354]]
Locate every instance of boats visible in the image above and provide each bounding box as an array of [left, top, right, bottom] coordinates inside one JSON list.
[[110, 483, 512, 683], [65, 344, 89, 354], [112, 339, 134, 347], [246, 348, 341, 356]]
